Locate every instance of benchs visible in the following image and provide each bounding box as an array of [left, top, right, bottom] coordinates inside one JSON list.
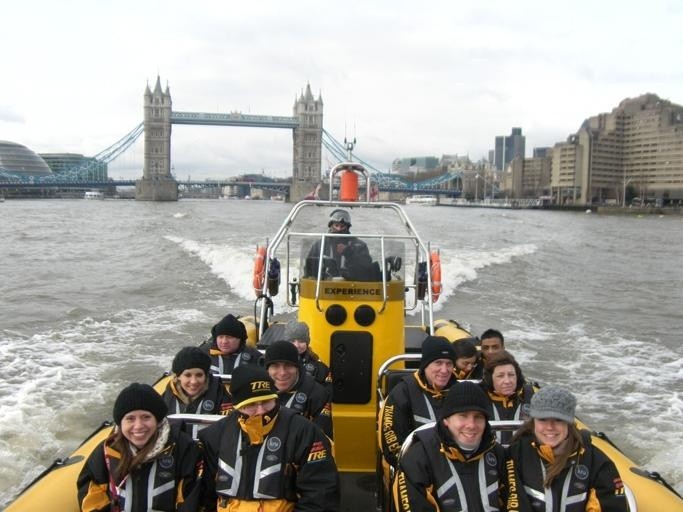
[[212, 373, 233, 393], [378, 353, 428, 407], [378, 379, 485, 492], [161, 415, 229, 480], [256, 350, 321, 363], [391, 421, 640, 512]]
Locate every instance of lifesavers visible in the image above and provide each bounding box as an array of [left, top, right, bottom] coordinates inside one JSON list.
[[253, 244, 267, 298], [433, 253, 442, 302], [268, 258, 281, 296], [415, 262, 428, 302]]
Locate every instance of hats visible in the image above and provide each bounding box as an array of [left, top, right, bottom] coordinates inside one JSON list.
[[283, 320, 310, 345], [171, 346, 212, 377], [328, 208, 352, 227], [211, 313, 248, 340], [440, 381, 490, 421], [263, 340, 300, 370], [528, 384, 578, 425], [113, 381, 169, 426], [229, 364, 279, 411], [420, 335, 457, 371]]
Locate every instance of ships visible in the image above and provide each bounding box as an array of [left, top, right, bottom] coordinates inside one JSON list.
[[1, 134, 683, 512]]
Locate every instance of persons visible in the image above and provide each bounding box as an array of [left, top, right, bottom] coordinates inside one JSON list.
[[196, 366, 339, 512], [77, 383, 217, 512], [480, 328, 505, 378], [266, 341, 332, 440], [161, 348, 233, 439], [451, 340, 477, 375], [284, 321, 331, 388], [508, 388, 630, 512], [391, 380, 524, 511], [304, 208, 371, 278], [379, 337, 462, 512], [480, 353, 540, 449], [203, 314, 265, 394]]
